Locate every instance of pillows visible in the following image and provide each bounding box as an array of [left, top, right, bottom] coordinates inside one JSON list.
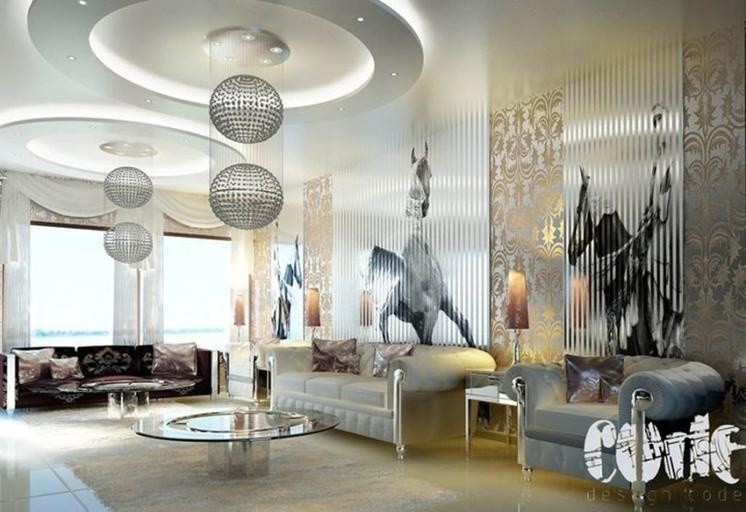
[[373, 342, 412, 378], [565, 355, 626, 405], [48, 356, 85, 380], [12, 348, 54, 384], [313, 337, 356, 371]]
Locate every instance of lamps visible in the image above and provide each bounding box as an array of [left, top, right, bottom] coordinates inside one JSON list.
[[508, 270, 528, 363], [307, 288, 320, 342], [203, 26, 291, 231], [233, 296, 245, 343], [569, 273, 591, 330], [99, 140, 158, 264], [358, 289, 374, 327]]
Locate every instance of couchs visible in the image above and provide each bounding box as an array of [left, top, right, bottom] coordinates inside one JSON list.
[[1, 344, 220, 406], [503, 354, 726, 510], [151, 342, 198, 378], [268, 343, 497, 456]]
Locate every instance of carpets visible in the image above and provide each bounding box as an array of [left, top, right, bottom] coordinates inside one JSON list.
[[62, 432, 468, 512]]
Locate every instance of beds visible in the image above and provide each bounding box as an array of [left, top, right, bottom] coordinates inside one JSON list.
[[132, 406, 340, 476]]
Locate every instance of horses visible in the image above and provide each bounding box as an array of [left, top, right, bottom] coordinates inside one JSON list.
[[365, 140, 477, 349], [567, 165, 684, 360]]
